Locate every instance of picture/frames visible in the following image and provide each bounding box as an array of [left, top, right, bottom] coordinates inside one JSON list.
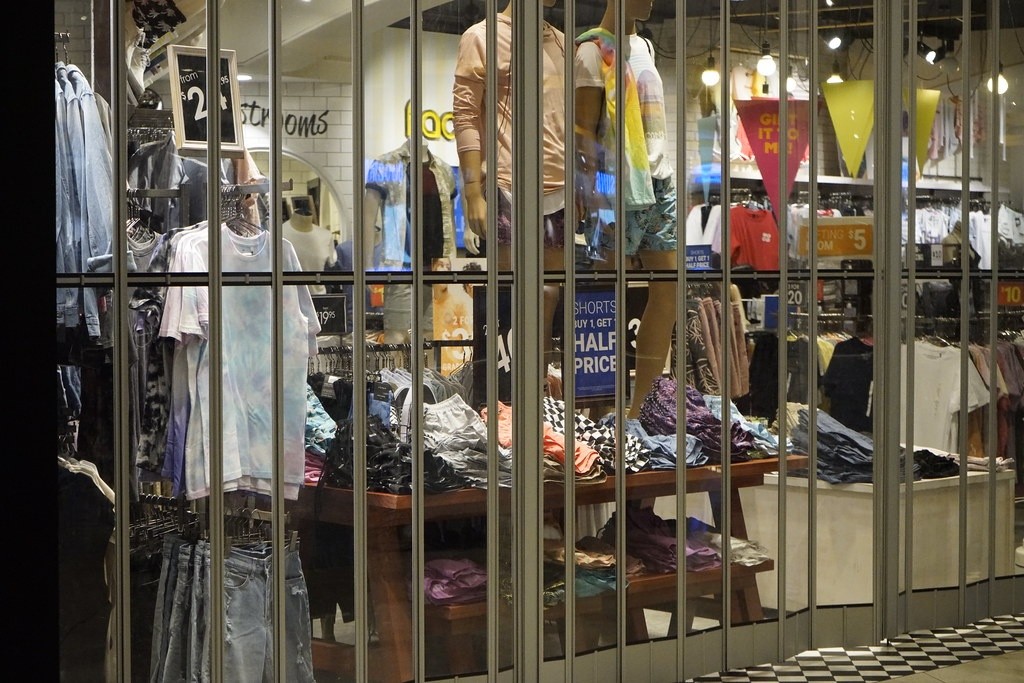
[[166, 45, 245, 159]]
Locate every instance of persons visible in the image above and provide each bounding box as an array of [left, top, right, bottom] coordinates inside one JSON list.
[[577, 0, 684, 436], [364, 132, 454, 363], [456, 1, 575, 422], [279, 208, 337, 296]]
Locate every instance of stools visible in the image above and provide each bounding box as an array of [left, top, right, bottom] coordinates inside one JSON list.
[[425, 561, 604, 676], [609, 556, 774, 647]]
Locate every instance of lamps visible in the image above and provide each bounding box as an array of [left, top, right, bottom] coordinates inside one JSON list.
[[702, 1, 721, 85], [818, 26, 840, 49], [757, 0, 776, 76], [986, 61, 1008, 95], [828, 49, 842, 84], [917, 40, 935, 62]]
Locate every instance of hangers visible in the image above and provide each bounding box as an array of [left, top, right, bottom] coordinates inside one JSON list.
[[54, 34, 61, 61], [60, 424, 77, 460], [686, 277, 717, 298], [125, 189, 265, 245], [127, 493, 299, 587], [791, 312, 845, 335], [705, 190, 1007, 214], [127, 124, 172, 156], [306, 341, 476, 373], [64, 33, 71, 66], [928, 312, 1024, 345]]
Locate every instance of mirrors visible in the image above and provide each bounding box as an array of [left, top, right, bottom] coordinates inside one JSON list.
[[249, 147, 350, 250]]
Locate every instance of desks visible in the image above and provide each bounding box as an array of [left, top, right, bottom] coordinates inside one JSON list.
[[290, 443, 808, 683]]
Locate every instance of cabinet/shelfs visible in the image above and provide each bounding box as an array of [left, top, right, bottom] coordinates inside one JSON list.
[[608, 445, 1013, 615], [687, 166, 1009, 339]]
